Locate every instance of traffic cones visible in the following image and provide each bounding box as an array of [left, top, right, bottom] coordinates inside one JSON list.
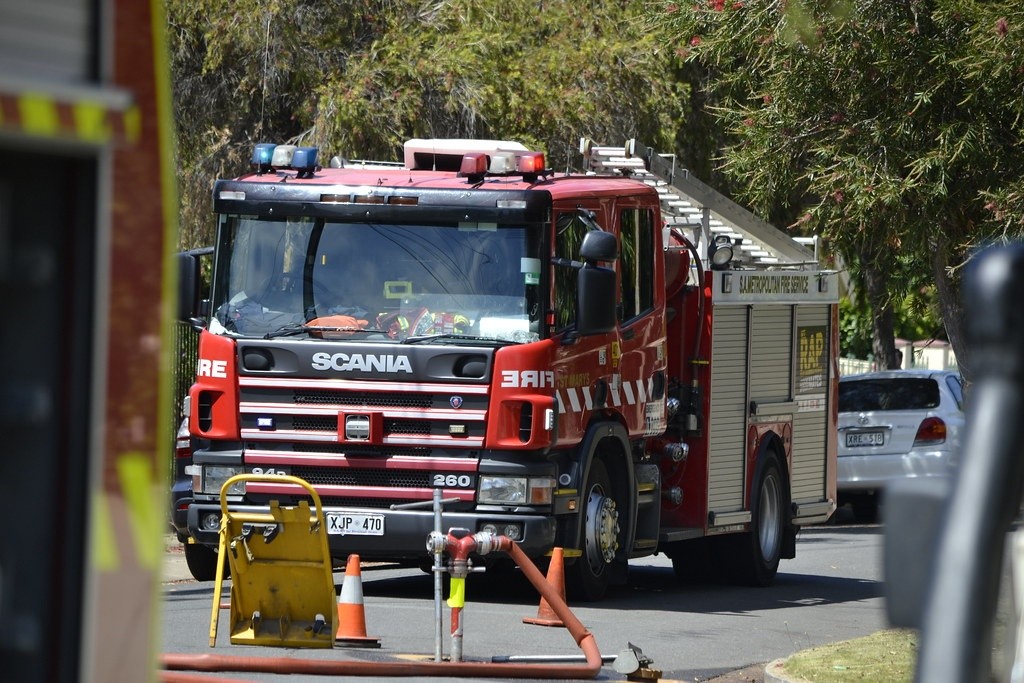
[[522, 548, 568, 627], [333, 554, 383, 648]]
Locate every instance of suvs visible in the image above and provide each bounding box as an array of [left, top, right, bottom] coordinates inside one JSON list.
[[830, 369, 967, 524]]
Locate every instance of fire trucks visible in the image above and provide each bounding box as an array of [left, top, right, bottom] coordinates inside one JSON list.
[[167, 134, 839, 606], [0, 0, 178, 683]]
